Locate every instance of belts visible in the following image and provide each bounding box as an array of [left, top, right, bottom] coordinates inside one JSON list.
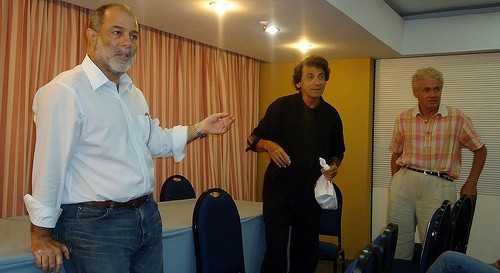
[[402, 164, 454, 184], [85, 196, 148, 209]]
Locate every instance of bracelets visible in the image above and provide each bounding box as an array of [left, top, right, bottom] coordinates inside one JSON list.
[[194, 123, 206, 139]]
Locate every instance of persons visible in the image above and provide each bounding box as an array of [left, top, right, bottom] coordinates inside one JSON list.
[[244, 54, 346, 273], [23, 1, 236, 273], [386, 67, 487, 261]]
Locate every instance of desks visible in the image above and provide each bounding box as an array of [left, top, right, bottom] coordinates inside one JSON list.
[[0, 198, 266, 273]]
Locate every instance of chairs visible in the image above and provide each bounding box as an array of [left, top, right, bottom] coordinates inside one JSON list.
[[160, 175, 195, 203], [319, 181, 474, 273], [192, 186, 246, 273]]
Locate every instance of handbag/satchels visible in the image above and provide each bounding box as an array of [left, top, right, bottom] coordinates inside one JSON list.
[[313, 157, 338, 211]]
